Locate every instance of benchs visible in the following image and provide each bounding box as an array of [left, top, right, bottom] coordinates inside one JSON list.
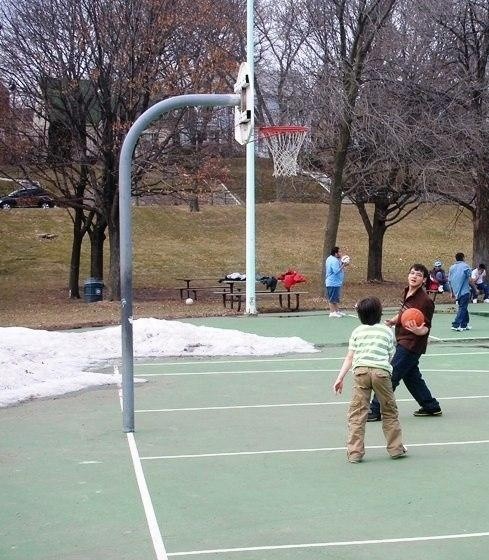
[[174, 286, 309, 311], [425, 275, 485, 303]]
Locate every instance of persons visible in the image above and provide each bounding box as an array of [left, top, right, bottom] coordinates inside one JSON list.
[[334, 296, 409, 464], [323, 247, 352, 317], [364, 263, 441, 423], [429, 261, 447, 293], [449, 253, 479, 332], [471, 264, 489, 304]]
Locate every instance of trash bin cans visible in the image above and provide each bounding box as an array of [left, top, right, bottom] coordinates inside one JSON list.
[[188, 195, 199, 212], [84, 282, 104, 303]]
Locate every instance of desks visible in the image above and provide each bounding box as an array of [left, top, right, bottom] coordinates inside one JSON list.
[[176, 277, 307, 309]]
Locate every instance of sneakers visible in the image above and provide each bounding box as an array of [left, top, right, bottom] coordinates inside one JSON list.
[[451, 325, 472, 331], [329, 311, 345, 318], [366, 413, 381, 422], [413, 406, 443, 416], [392, 446, 407, 459], [348, 456, 362, 463]]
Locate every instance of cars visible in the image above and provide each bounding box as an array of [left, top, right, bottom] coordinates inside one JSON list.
[[0, 188, 55, 209]]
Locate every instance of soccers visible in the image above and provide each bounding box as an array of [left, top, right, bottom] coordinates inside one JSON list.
[[341, 255, 350, 263], [186, 298, 193, 305]]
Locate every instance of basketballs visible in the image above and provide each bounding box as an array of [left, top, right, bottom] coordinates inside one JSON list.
[[401, 308, 425, 328]]
